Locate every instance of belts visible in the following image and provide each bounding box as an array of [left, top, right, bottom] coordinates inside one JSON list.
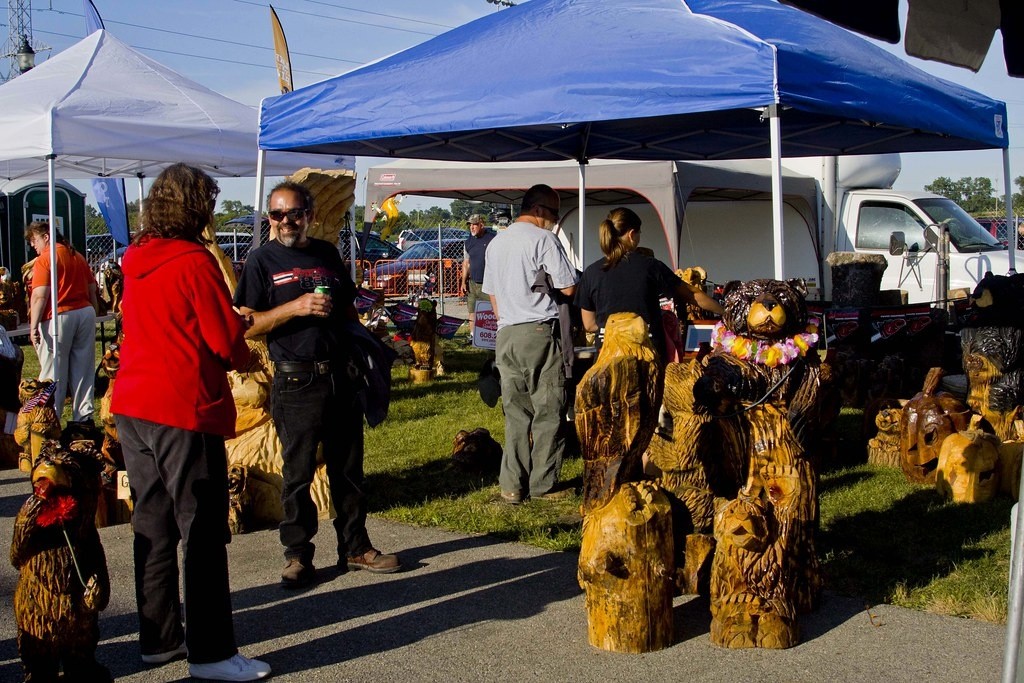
[[274, 361, 344, 374]]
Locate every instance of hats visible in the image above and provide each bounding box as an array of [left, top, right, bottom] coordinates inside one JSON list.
[[467, 214, 486, 225]]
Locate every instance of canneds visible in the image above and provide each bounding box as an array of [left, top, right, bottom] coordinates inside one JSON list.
[[314, 286, 331, 296]]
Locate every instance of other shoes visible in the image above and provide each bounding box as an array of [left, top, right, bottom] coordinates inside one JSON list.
[[500, 491, 521, 504], [531, 485, 577, 501]]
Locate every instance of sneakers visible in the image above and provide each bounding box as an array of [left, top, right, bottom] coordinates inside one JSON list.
[[189, 652, 272, 681], [280, 556, 317, 587], [142, 639, 187, 662], [336, 548, 399, 573]]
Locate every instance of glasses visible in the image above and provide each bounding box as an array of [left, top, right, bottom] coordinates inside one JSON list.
[[267, 208, 304, 221], [532, 204, 559, 216]]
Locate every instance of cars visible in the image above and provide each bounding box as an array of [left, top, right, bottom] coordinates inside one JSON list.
[[211, 228, 469, 298], [974, 215, 1024, 251]]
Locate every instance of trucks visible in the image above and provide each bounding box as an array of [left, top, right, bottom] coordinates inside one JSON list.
[[828, 187, 1023, 309]]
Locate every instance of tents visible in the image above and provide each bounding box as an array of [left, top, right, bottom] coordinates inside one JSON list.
[[0, 29, 356, 423], [232, 0, 1018, 283]]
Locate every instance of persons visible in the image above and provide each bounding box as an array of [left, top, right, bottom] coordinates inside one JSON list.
[[577, 207, 725, 370], [481, 183, 578, 505], [110, 161, 270, 682], [459, 214, 502, 345], [232, 181, 404, 586], [1017, 221, 1024, 250], [24, 221, 98, 423]]
